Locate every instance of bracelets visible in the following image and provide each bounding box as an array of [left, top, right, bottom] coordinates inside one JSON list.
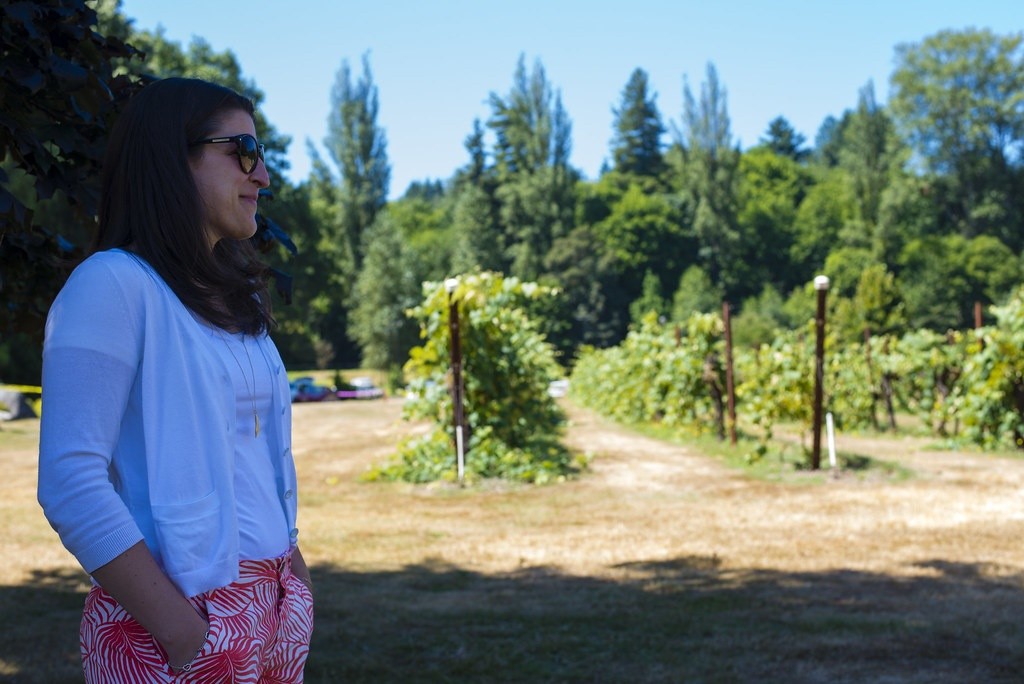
[[298, 576, 313, 588]]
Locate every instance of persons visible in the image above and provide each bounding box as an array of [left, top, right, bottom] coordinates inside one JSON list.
[[38, 76, 314, 684]]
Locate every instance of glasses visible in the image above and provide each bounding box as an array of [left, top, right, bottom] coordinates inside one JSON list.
[[191, 133, 265, 175]]
